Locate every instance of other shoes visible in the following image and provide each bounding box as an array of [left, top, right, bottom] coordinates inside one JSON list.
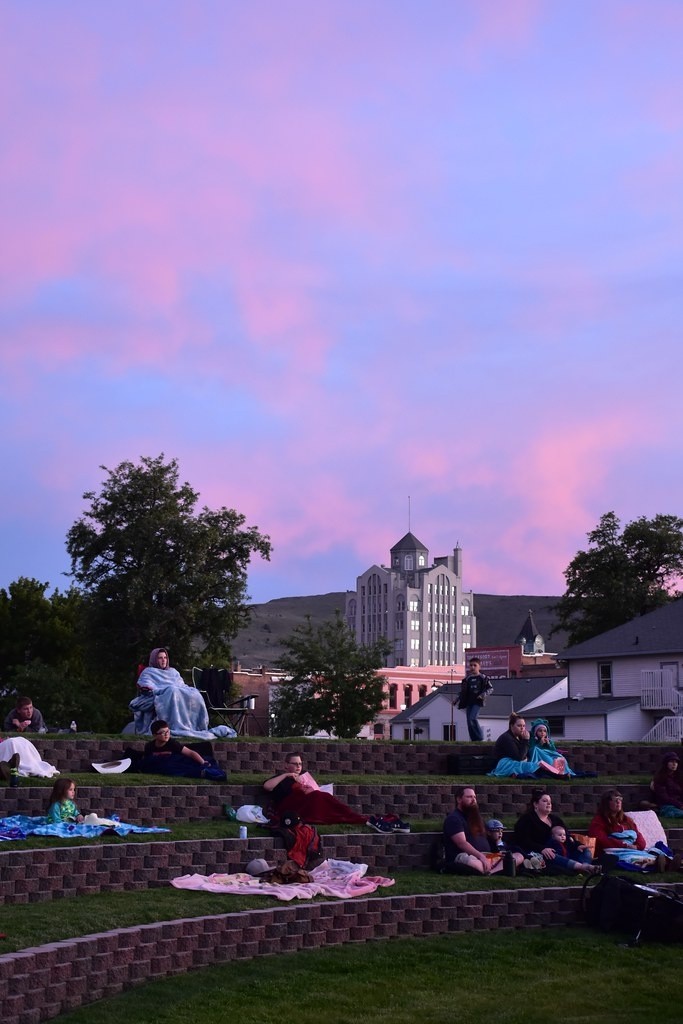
[[668, 854, 681, 871], [583, 863, 602, 874], [655, 855, 665, 873]]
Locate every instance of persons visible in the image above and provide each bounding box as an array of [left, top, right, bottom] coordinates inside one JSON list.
[[4, 696, 48, 734], [640, 752, 683, 811], [452, 658, 494, 742], [48, 778, 84, 825], [492, 713, 571, 781], [143, 719, 212, 766], [441, 788, 602, 875], [260, 750, 302, 804], [588, 789, 681, 874], [136, 647, 210, 734]]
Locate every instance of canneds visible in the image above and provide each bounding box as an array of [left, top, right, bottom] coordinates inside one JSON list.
[[239, 825, 247, 839]]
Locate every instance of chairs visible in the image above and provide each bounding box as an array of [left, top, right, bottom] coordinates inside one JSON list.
[[134, 664, 267, 737]]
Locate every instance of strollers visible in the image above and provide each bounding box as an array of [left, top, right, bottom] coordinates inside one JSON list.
[[579, 871, 683, 947]]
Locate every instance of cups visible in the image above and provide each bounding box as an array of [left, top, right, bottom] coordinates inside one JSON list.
[[240, 826, 248, 839]]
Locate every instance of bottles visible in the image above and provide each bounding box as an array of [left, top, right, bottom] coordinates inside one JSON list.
[[70, 721, 78, 732]]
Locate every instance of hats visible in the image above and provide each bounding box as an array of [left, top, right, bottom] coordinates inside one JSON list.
[[247, 858, 275, 875], [485, 820, 507, 829], [534, 724, 547, 734], [663, 752, 680, 762], [92, 758, 131, 774]]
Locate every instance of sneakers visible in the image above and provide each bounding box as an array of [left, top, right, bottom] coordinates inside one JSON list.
[[559, 772, 570, 780], [388, 820, 410, 833], [366, 815, 393, 833]]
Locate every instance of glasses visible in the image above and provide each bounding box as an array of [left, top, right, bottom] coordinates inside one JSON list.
[[154, 729, 170, 736], [287, 762, 303, 766], [609, 796, 623, 802]]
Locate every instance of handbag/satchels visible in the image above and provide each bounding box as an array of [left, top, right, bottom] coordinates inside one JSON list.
[[236, 805, 270, 823]]
